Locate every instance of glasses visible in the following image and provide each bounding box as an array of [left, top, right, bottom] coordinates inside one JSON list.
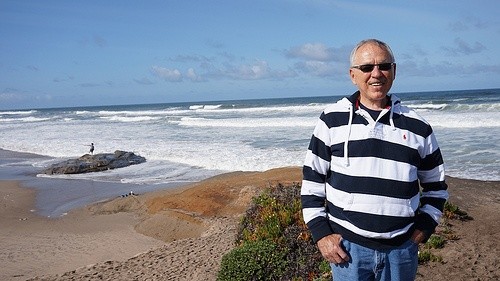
[[350, 63, 392, 74]]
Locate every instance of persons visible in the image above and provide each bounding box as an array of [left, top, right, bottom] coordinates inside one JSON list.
[[301, 39, 449, 281]]
